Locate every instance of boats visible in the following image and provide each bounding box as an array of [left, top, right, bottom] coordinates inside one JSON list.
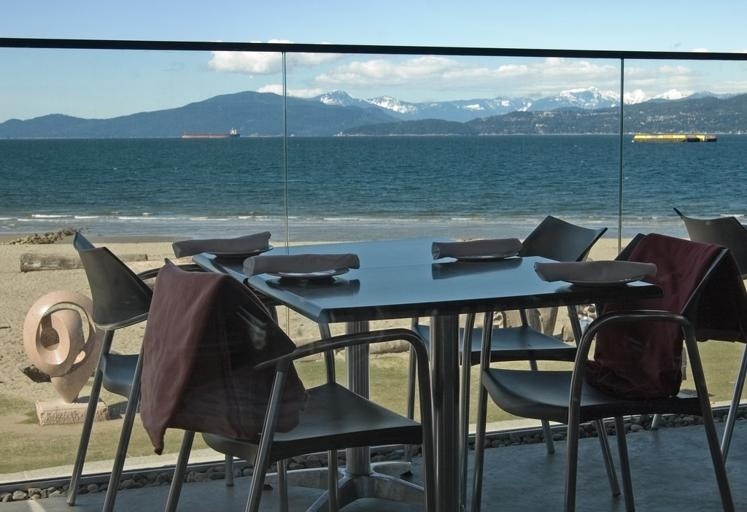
[[181, 128, 240, 138], [632, 133, 717, 142]]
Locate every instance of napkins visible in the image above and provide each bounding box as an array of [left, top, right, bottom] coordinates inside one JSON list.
[[170, 228, 273, 259], [242, 251, 362, 281], [531, 258, 658, 287], [431, 237, 524, 263]]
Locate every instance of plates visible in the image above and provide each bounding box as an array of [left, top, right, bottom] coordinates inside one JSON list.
[[265, 267, 350, 283], [454, 251, 520, 263], [564, 274, 646, 290], [207, 243, 274, 259]]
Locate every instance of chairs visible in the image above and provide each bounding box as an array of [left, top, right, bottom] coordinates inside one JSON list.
[[155, 258, 443, 512], [401, 212, 609, 510], [66, 230, 237, 512], [470, 230, 735, 511], [650, 206, 747, 467]]
